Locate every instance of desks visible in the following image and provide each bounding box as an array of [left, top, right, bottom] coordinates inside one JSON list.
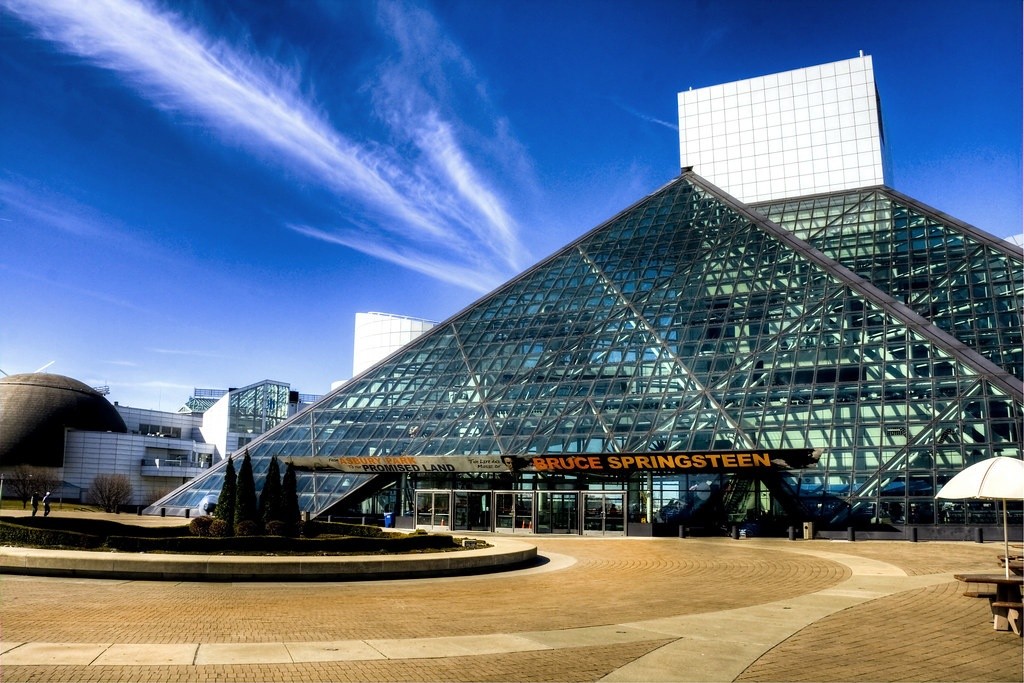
[[954, 574, 1024, 631]]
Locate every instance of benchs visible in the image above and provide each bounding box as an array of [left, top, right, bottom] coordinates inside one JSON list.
[[962, 592, 1024, 631]]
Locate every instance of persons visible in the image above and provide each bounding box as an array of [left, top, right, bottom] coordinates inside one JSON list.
[[610, 504, 617, 530], [42, 492, 51, 517], [31, 489, 39, 516]]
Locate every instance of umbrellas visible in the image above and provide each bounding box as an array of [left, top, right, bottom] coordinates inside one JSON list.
[[934, 456, 1024, 579]]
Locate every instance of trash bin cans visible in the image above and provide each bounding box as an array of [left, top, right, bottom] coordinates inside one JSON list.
[[384, 512, 395, 528]]
